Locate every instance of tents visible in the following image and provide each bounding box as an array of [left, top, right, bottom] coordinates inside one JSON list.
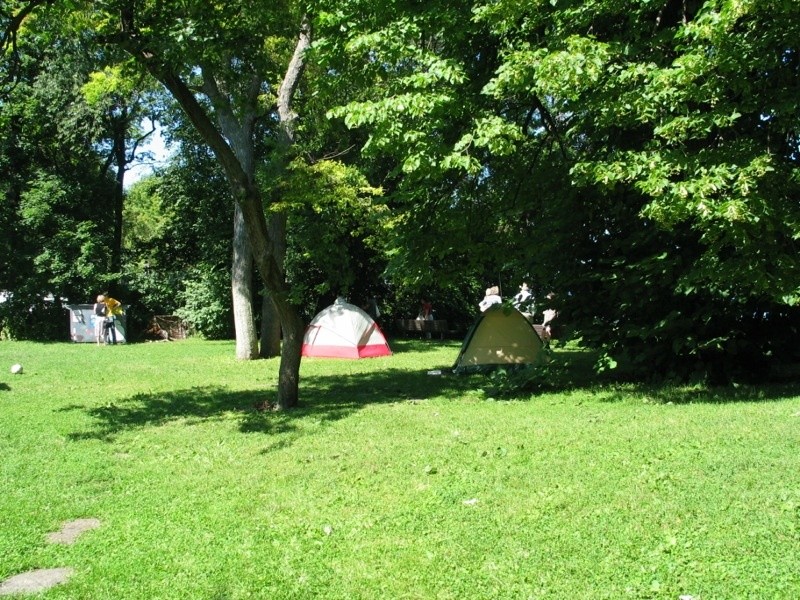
[[448, 305, 561, 375], [299, 296, 393, 360]]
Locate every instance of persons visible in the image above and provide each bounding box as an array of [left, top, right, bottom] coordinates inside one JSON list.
[[360, 295, 380, 323], [92, 291, 121, 345], [420, 297, 433, 341], [477, 281, 557, 349]]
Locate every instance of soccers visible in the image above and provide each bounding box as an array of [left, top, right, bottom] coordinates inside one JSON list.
[[11, 364, 23, 374]]
